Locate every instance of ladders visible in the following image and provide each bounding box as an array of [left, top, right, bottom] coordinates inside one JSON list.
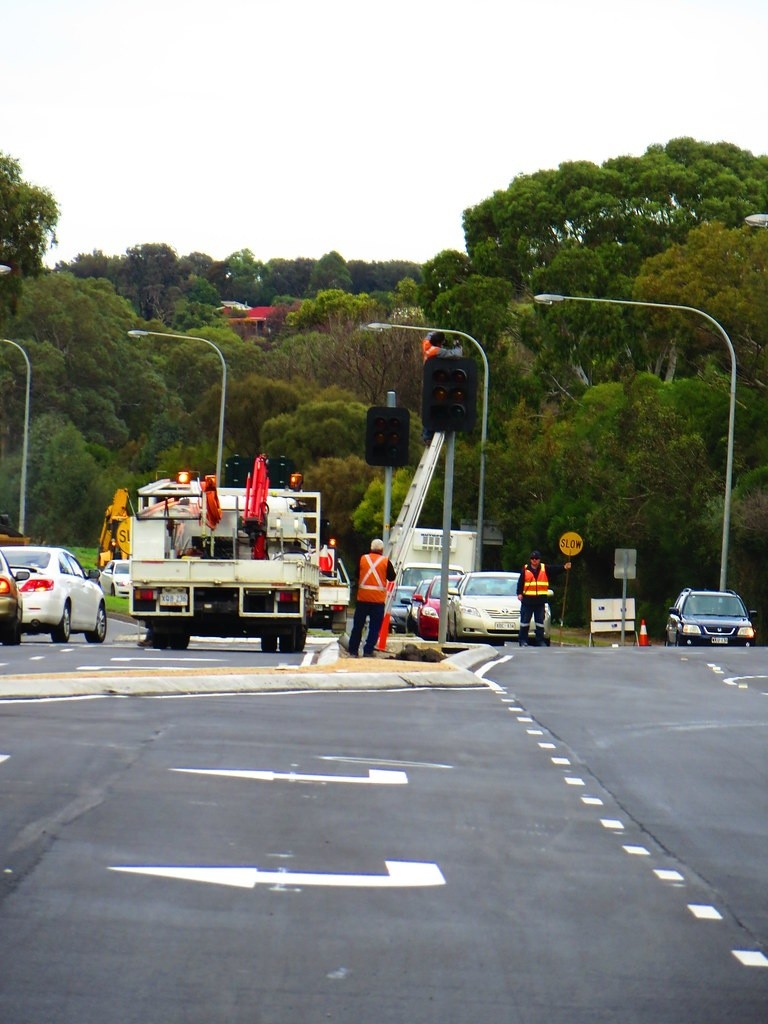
[[356, 432, 445, 657]]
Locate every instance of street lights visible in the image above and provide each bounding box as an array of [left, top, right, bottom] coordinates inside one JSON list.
[[533, 293, 736, 592], [363, 322, 489, 573], [126, 329, 227, 487]]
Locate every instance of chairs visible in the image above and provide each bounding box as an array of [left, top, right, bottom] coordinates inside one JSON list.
[[472, 584, 486, 594]]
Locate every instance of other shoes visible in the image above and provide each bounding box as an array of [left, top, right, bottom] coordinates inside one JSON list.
[[348, 649, 358, 657], [519, 641, 528, 646], [363, 652, 377, 657]]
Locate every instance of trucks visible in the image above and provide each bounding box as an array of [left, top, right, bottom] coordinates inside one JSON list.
[[127, 453, 352, 653], [383, 527, 478, 634]]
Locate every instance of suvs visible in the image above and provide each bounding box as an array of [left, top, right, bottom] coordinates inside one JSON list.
[[663, 587, 758, 647]]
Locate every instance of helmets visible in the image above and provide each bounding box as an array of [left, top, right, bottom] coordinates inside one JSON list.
[[371, 539, 385, 551], [529, 551, 540, 559]]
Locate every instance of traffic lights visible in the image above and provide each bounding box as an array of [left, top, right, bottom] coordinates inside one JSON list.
[[366, 405, 409, 468], [328, 537, 337, 549], [422, 355, 478, 432]]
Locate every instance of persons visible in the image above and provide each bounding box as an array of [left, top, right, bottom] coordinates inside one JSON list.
[[250, 529, 270, 560], [421, 332, 462, 448], [516, 550, 571, 648], [347, 539, 396, 659]]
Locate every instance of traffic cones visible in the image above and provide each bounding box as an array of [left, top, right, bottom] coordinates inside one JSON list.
[[635, 618, 652, 646]]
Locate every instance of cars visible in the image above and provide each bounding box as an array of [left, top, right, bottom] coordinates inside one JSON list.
[[95, 559, 130, 599], [448, 571, 554, 646], [412, 574, 464, 641], [400, 579, 433, 635], [0, 546, 108, 643], [387, 585, 417, 634], [0, 550, 31, 646]]
